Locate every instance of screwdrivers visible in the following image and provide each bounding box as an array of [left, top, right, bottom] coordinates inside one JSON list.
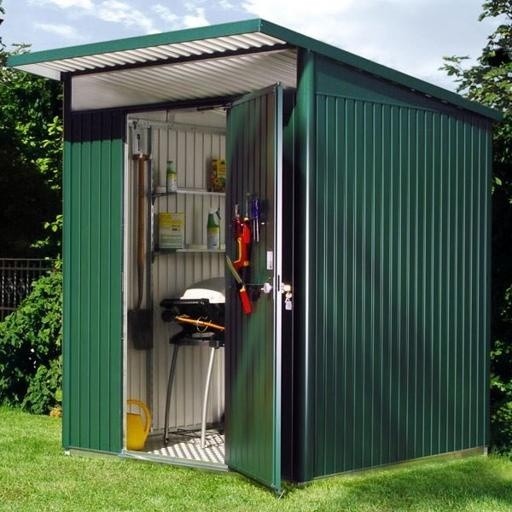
[[251, 198, 261, 244]]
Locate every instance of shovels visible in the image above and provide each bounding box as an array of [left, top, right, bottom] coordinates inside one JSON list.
[[127, 151, 154, 351]]
